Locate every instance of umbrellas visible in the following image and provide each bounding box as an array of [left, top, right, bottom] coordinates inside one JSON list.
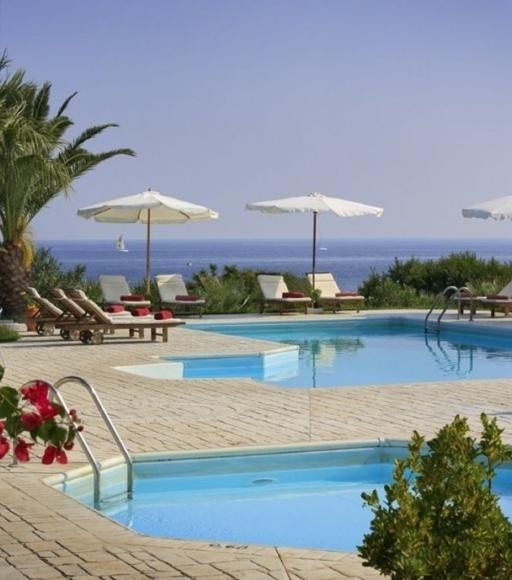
[[245, 190, 384, 287], [462, 193, 512, 225], [77, 185, 220, 296]]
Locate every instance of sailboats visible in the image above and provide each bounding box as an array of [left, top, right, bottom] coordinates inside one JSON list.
[[116, 234, 128, 253]]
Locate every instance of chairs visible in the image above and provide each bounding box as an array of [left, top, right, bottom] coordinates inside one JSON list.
[[454, 280, 512, 317], [305, 272, 365, 314], [155, 275, 205, 318], [255, 272, 312, 315], [26, 287, 185, 344], [96, 275, 151, 308]]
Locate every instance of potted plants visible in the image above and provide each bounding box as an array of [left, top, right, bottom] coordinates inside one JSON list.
[[20, 247, 85, 331], [310, 289, 322, 308]]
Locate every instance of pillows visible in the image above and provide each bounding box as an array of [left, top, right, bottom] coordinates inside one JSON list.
[[336, 292, 357, 296], [175, 295, 198, 301], [282, 292, 305, 298], [486, 294, 508, 299]]
